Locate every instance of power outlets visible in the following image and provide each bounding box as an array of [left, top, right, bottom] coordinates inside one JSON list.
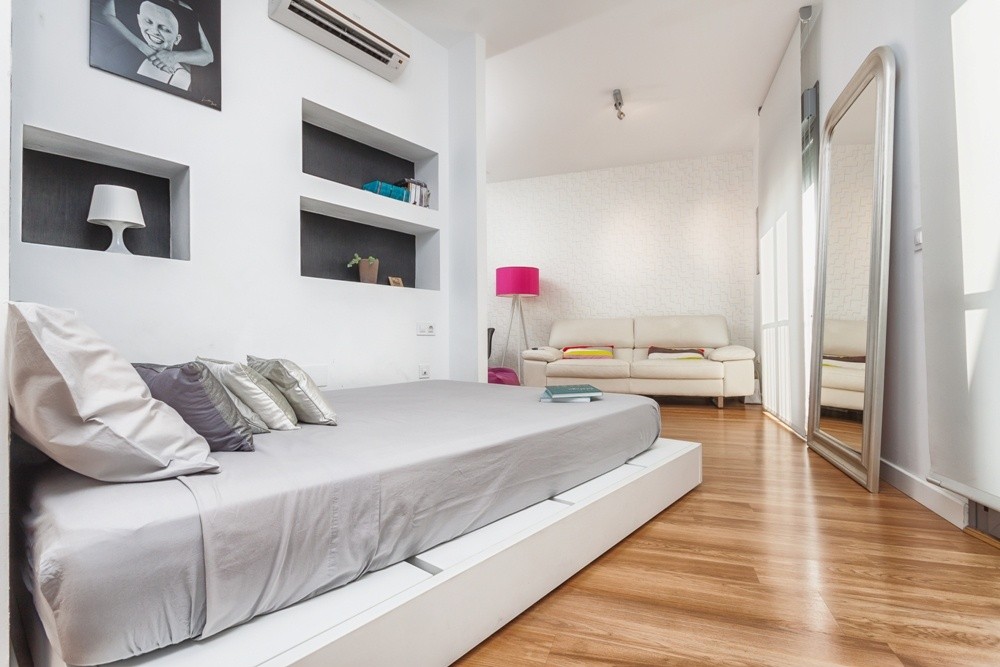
[[417, 322, 436, 336]]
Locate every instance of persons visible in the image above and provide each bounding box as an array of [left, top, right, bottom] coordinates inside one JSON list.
[[104, 0, 214, 92]]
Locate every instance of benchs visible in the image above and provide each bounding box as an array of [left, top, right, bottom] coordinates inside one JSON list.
[[524, 315, 755, 408]]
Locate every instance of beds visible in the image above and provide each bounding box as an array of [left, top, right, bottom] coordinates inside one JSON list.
[[0, 380, 703, 667]]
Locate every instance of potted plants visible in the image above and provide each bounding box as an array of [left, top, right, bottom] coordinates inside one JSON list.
[[347, 253, 379, 284]]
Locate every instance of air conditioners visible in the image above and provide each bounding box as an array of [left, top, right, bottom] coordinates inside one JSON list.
[[270, 0, 412, 82]]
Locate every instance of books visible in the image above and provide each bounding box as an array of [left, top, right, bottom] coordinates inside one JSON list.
[[540, 384, 602, 404]]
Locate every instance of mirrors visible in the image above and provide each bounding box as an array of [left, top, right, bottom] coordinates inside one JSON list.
[[807, 44, 895, 493]]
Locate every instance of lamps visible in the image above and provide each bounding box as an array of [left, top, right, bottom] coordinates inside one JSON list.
[[496, 266, 540, 383], [613, 89, 625, 120], [87, 184, 146, 255]]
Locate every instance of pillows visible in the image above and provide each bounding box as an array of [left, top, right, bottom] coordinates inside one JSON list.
[[8, 302, 337, 483], [562, 346, 614, 359], [648, 346, 704, 359]]
